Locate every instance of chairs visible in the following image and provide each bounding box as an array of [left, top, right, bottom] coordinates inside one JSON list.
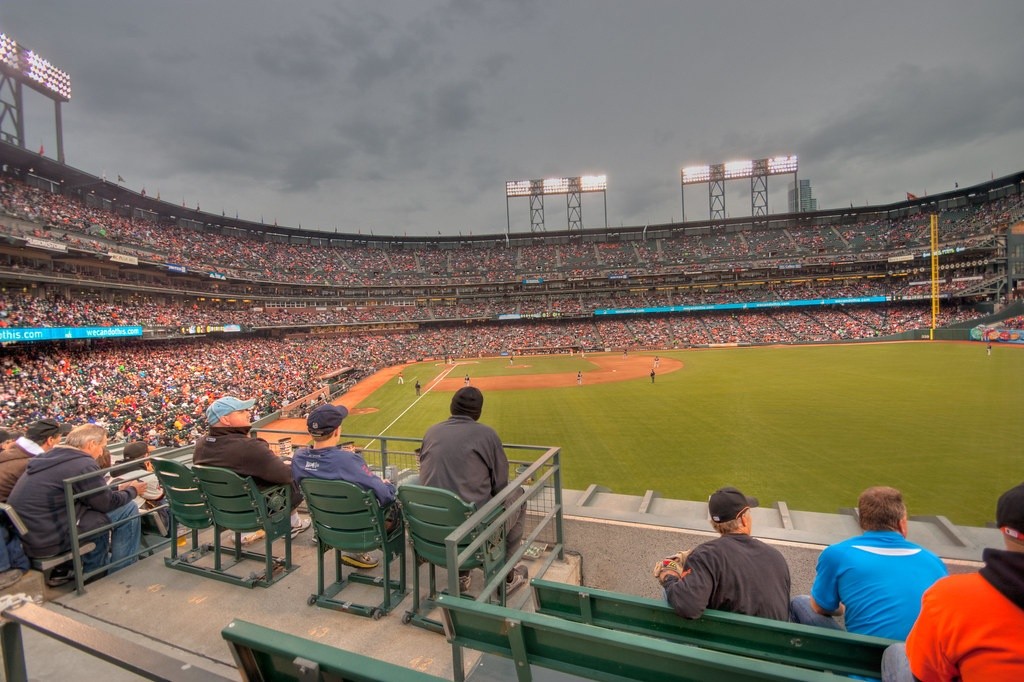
[[147, 457, 241, 572], [0, 502, 97, 572], [139, 508, 172, 558], [396, 485, 506, 616], [299, 477, 406, 611], [190, 464, 294, 581]]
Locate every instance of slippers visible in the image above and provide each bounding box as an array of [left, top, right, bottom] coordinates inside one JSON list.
[[281, 517, 311, 540]]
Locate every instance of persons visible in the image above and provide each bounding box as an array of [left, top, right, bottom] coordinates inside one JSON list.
[[652, 486, 796, 670], [289, 403, 406, 570], [102, 441, 178, 539], [881, 481, 1024, 682], [0, 418, 61, 529], [789, 485, 949, 682], [419, 386, 529, 602], [0, 155, 1024, 466], [192, 395, 312, 545], [6, 422, 149, 587]]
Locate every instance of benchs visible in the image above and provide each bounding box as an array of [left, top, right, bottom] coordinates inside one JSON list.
[[220, 577, 906, 682]]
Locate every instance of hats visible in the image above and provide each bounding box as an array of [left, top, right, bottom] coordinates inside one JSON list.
[[997, 483, 1024, 541], [451, 387, 483, 416], [709, 486, 760, 524], [307, 405, 348, 436], [123, 442, 156, 461], [205, 396, 256, 426], [0, 428, 24, 446], [27, 419, 72, 439]]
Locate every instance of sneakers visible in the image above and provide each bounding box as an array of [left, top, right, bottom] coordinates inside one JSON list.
[[459, 571, 470, 595], [497, 565, 528, 597]]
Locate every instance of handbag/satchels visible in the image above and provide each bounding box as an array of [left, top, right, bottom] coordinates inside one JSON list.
[[6, 538, 30, 576]]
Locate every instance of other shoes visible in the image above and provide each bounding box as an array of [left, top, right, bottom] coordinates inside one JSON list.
[[0, 569, 23, 589], [47, 559, 83, 586], [83, 569, 108, 586], [343, 552, 379, 568]]
